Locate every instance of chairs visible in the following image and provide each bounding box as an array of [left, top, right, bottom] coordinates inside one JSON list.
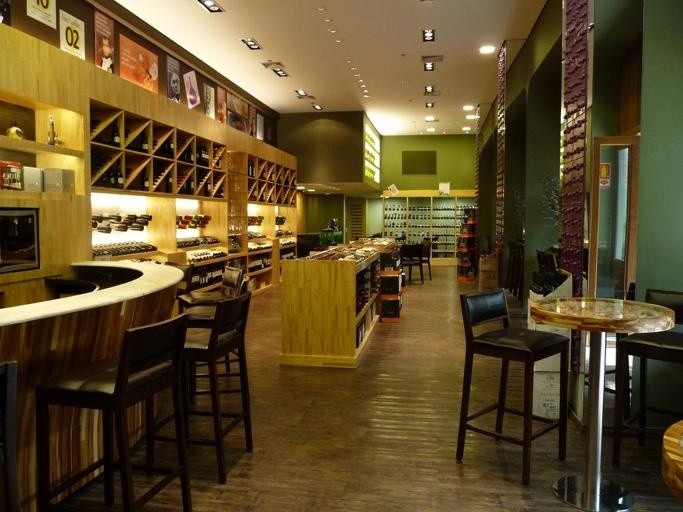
[[457, 288, 569, 484], [613, 290, 683, 468]]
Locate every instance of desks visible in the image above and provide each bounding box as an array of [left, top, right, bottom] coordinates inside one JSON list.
[[660, 419, 682, 501], [530, 297, 675, 512]]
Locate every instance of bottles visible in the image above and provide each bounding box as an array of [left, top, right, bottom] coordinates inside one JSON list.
[[141, 138, 149, 154], [47, 116, 64, 146], [142, 173, 149, 192], [181, 175, 195, 195], [187, 147, 194, 164], [101, 165, 124, 189], [165, 171, 173, 193], [165, 138, 175, 159], [6, 121, 24, 140], [217, 176, 224, 198], [199, 145, 209, 167], [91, 213, 298, 292], [205, 179, 211, 197], [113, 127, 121, 148], [249, 160, 255, 177]]
